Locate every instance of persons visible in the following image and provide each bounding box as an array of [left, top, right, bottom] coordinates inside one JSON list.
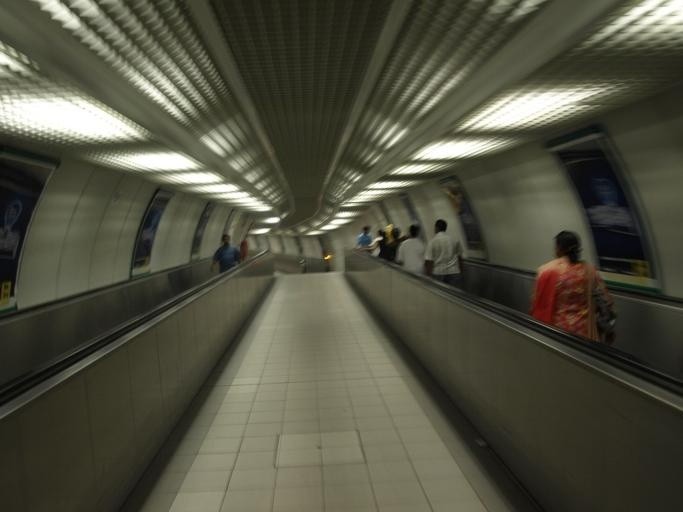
[[210, 233, 241, 273], [354, 218, 464, 287], [297, 254, 307, 273], [528, 228, 620, 347], [320, 251, 331, 271]]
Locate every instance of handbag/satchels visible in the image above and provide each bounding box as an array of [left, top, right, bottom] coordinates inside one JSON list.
[[596, 315, 617, 345]]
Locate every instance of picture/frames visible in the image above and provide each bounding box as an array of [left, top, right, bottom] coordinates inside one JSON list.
[[130, 186, 175, 277], [544, 122, 663, 295], [440, 175, 488, 261], [0, 145, 59, 313]]
